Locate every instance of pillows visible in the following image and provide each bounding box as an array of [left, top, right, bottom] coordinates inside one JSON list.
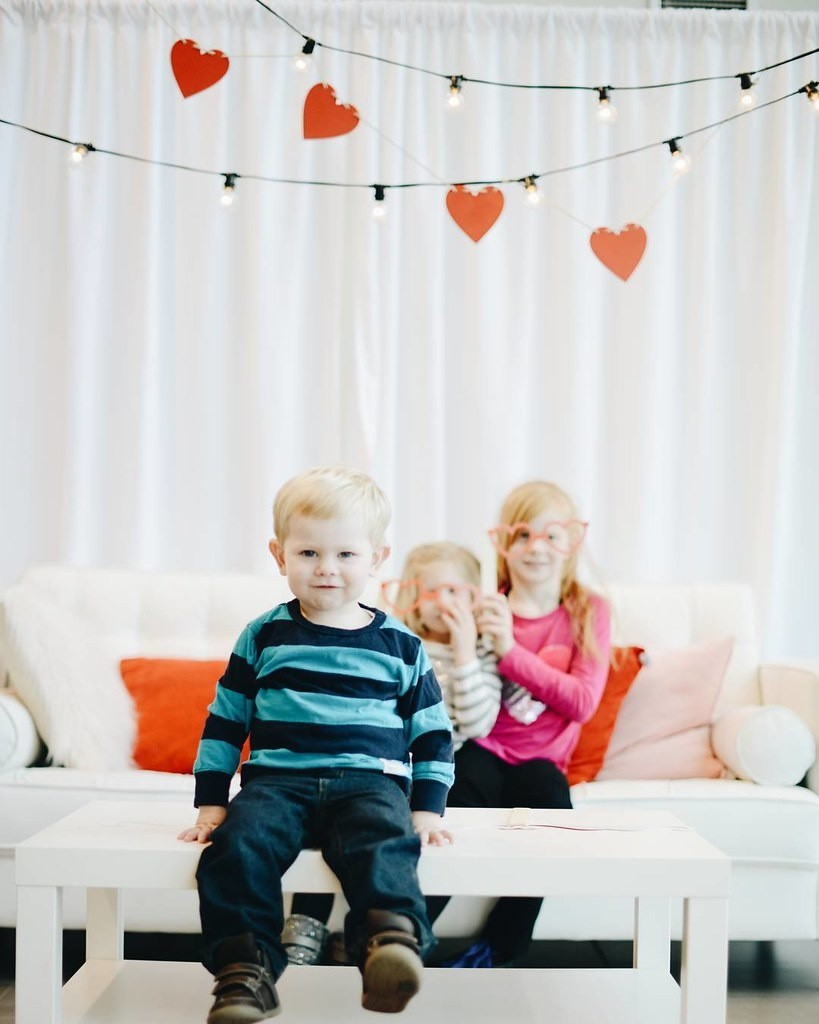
[[565, 644, 646, 789], [0, 574, 143, 771], [116, 657, 251, 774], [591, 614, 737, 783], [710, 702, 816, 786]]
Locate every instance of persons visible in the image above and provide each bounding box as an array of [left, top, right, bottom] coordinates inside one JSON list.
[[177, 465, 455, 1024], [412, 477, 614, 970], [276, 539, 503, 967]]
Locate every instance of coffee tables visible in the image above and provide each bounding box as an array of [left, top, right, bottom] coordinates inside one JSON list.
[[14, 792, 739, 1024]]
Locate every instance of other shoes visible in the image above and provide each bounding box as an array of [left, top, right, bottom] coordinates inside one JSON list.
[[281, 913, 330, 966], [362, 912, 423, 1013], [207, 964, 282, 1024]]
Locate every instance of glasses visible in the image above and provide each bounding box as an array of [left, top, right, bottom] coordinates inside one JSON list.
[[381, 579, 479, 615], [486, 518, 590, 561]]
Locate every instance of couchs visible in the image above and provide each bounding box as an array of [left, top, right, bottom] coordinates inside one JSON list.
[[0, 568, 818, 941]]
